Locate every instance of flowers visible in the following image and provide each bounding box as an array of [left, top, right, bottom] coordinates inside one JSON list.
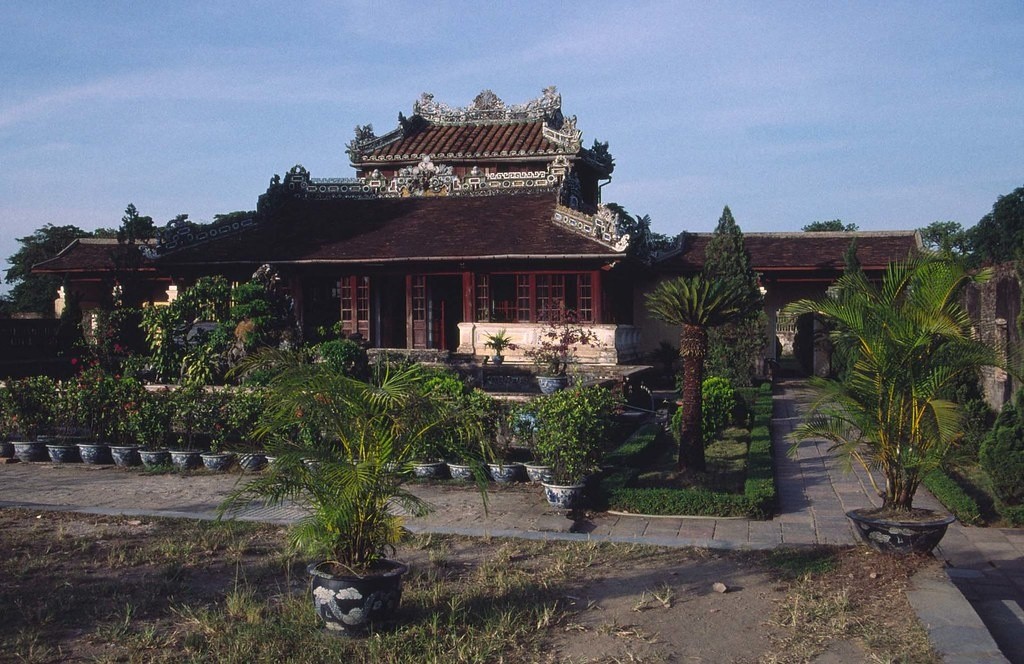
[[65, 320, 152, 424]]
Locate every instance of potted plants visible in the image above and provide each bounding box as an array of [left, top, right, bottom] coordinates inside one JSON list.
[[775, 236, 1012, 562], [746, 311, 773, 383], [0, 277, 634, 635]]
